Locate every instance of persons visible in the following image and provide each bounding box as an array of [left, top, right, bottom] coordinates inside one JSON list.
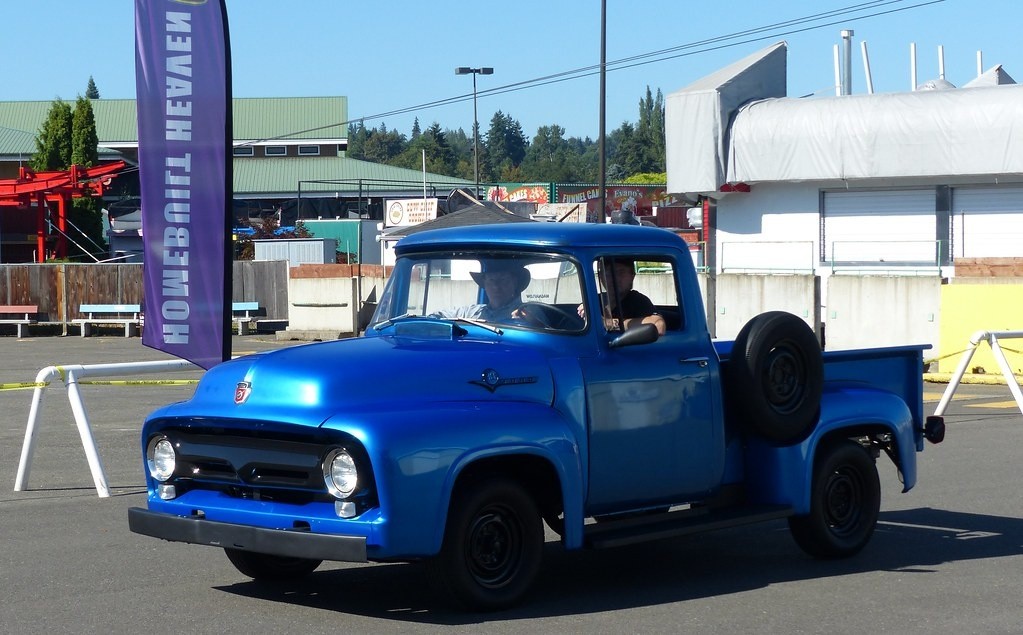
[[510, 256, 666, 334], [429, 260, 532, 322]]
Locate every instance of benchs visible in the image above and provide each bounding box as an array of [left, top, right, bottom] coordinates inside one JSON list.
[[72, 304, 140, 338], [0, 305, 38, 338], [545, 304, 681, 332], [231, 301, 258, 335]]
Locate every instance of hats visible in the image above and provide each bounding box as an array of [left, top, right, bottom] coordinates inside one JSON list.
[[469, 261, 531, 292]]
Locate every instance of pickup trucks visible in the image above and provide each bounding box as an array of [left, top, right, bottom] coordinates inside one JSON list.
[[128, 221, 945, 600]]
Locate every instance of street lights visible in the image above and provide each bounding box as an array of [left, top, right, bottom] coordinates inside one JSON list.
[[454, 66, 494, 201], [840, 30, 854, 96]]
